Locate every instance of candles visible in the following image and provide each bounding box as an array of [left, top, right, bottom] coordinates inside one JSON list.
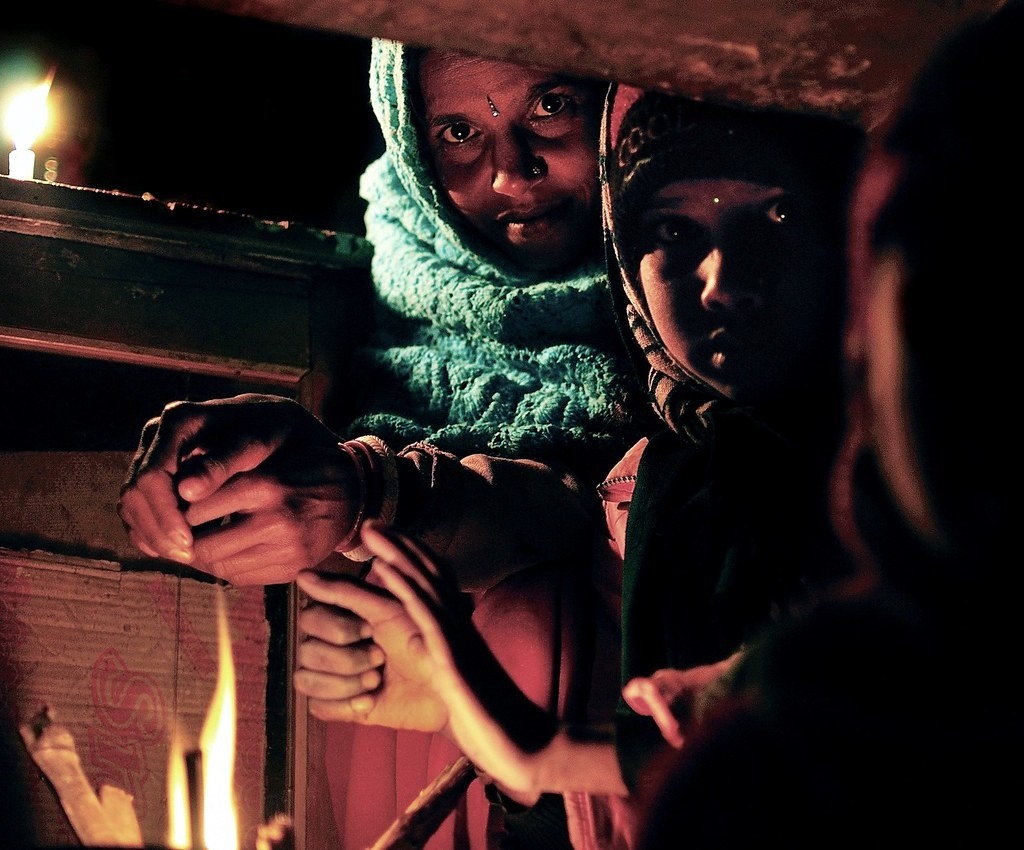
[[9, 149, 36, 182]]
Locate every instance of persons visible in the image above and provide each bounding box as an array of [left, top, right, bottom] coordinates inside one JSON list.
[[119, 40, 863, 812]]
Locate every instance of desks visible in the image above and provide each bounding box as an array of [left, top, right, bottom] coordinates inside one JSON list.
[[0, 174, 373, 850]]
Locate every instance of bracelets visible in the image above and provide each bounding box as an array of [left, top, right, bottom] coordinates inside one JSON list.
[[330, 435, 402, 565]]
